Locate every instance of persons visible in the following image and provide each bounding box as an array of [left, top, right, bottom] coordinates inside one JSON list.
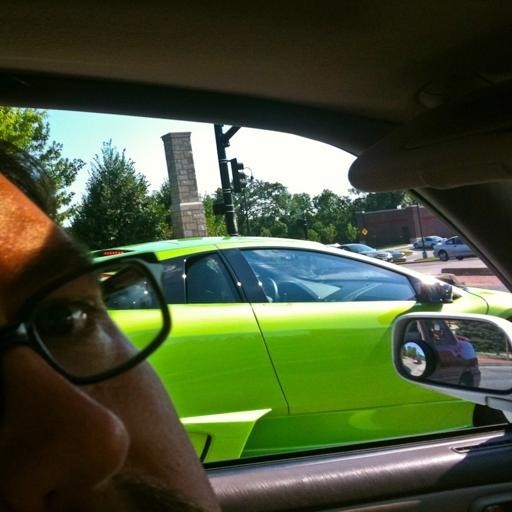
[[1, 136, 220, 512]]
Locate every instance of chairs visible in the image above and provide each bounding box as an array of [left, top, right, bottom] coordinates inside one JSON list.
[[162, 260, 220, 302]]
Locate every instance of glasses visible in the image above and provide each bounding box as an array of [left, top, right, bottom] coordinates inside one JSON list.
[[0, 251, 171, 427]]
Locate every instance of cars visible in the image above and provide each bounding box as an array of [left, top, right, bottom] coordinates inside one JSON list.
[[407, 320, 480, 388], [414, 235, 446, 250], [385, 250, 406, 262], [413, 359, 422, 364], [79, 237, 512, 464], [433, 234, 478, 261], [337, 243, 394, 264]]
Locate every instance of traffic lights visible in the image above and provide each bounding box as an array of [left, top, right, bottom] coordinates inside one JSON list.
[[231, 158, 248, 193]]
